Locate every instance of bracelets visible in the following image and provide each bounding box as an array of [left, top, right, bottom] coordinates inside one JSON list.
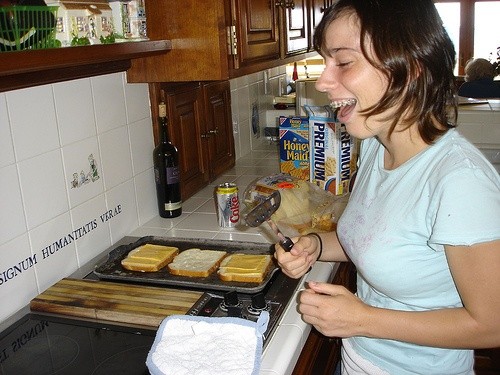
[[308, 232, 323, 261]]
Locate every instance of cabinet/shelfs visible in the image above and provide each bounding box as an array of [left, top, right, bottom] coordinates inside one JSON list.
[[128, 0, 311, 83], [294, 263, 357, 375], [150, 81, 239, 203], [309, 0, 340, 55]]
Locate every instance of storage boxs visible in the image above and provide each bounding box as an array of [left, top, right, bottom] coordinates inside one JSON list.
[[280, 103, 359, 196]]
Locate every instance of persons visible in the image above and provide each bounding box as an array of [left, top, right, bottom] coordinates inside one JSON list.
[[464, 58, 494, 83], [278, 0, 500, 375]]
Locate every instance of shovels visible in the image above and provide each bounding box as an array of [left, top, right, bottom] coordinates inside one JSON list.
[[245, 190, 311, 274]]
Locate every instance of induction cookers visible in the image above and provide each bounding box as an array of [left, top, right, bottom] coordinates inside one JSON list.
[[0, 235, 309, 375]]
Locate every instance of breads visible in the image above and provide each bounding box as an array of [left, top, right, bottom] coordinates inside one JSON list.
[[255, 173, 332, 233], [219, 253, 273, 283], [121, 244, 179, 272], [168, 248, 230, 277]]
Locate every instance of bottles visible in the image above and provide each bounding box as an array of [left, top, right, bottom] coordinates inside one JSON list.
[[152, 102, 182, 218]]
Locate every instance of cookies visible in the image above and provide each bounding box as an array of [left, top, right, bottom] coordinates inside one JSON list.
[[280, 153, 356, 191]]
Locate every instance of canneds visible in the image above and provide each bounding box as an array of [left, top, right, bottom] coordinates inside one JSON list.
[[216, 183, 241, 228]]
